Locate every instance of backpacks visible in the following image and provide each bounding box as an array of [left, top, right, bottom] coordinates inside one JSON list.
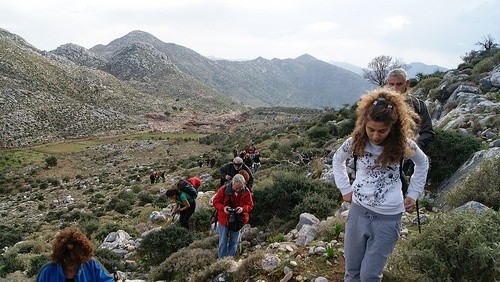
[[178, 177, 201, 198]]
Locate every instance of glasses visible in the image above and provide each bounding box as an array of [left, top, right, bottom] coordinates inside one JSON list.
[[233, 162, 242, 166]]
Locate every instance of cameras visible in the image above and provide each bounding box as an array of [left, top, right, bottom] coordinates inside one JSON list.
[[227, 208, 237, 222]]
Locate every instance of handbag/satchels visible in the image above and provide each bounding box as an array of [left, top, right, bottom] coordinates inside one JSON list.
[[227, 213, 244, 231]]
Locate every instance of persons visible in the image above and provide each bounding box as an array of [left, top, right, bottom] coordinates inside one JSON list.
[[220, 157, 253, 191], [150, 146, 261, 184], [212, 174, 253, 259], [36, 227, 122, 282], [332, 87, 429, 282], [387, 68, 436, 176], [166, 180, 197, 231]]
